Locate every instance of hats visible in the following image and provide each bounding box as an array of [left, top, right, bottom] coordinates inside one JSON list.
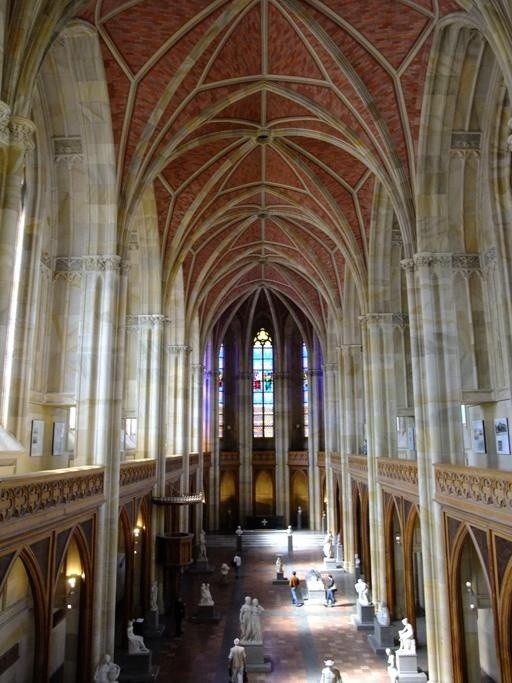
[[324, 660, 334, 666]]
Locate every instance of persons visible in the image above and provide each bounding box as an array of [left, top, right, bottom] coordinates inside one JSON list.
[[91, 654, 120, 683], [319, 659, 343, 683], [199, 582, 212, 605], [385, 617, 415, 683], [288, 570, 301, 606], [354, 554, 361, 568], [286, 525, 293, 536], [276, 557, 283, 573], [323, 575, 337, 607], [355, 578, 371, 606], [240, 595, 265, 642], [126, 618, 151, 656], [198, 529, 207, 560], [324, 533, 334, 558], [150, 579, 159, 609], [235, 525, 244, 536], [218, 552, 242, 584], [228, 638, 247, 683]]
